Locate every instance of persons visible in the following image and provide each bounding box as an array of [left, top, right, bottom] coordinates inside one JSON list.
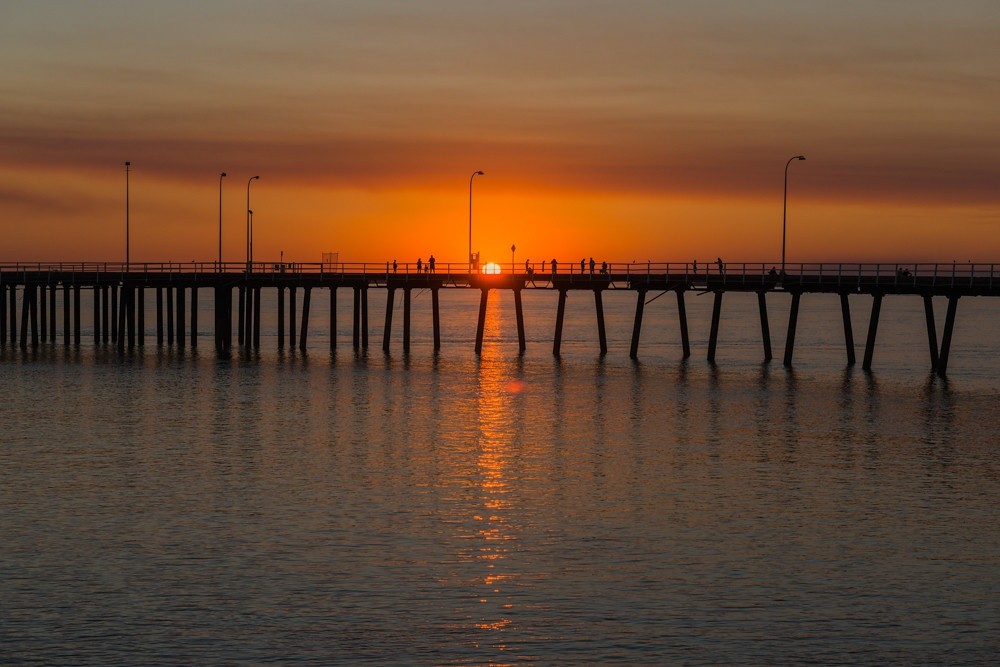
[[393, 260, 397, 274], [715, 258, 723, 274], [768, 266, 776, 275], [551, 259, 557, 274], [895, 268, 912, 277], [590, 257, 596, 274], [417, 255, 435, 274], [599, 262, 608, 274], [581, 258, 585, 274], [525, 258, 533, 274]]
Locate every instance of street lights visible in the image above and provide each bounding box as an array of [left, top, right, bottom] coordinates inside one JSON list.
[[125, 160, 131, 272], [468, 171, 484, 274], [246, 175, 261, 272], [218, 171, 227, 273], [248, 210, 254, 274], [782, 154, 805, 274], [511, 244, 516, 274]]
